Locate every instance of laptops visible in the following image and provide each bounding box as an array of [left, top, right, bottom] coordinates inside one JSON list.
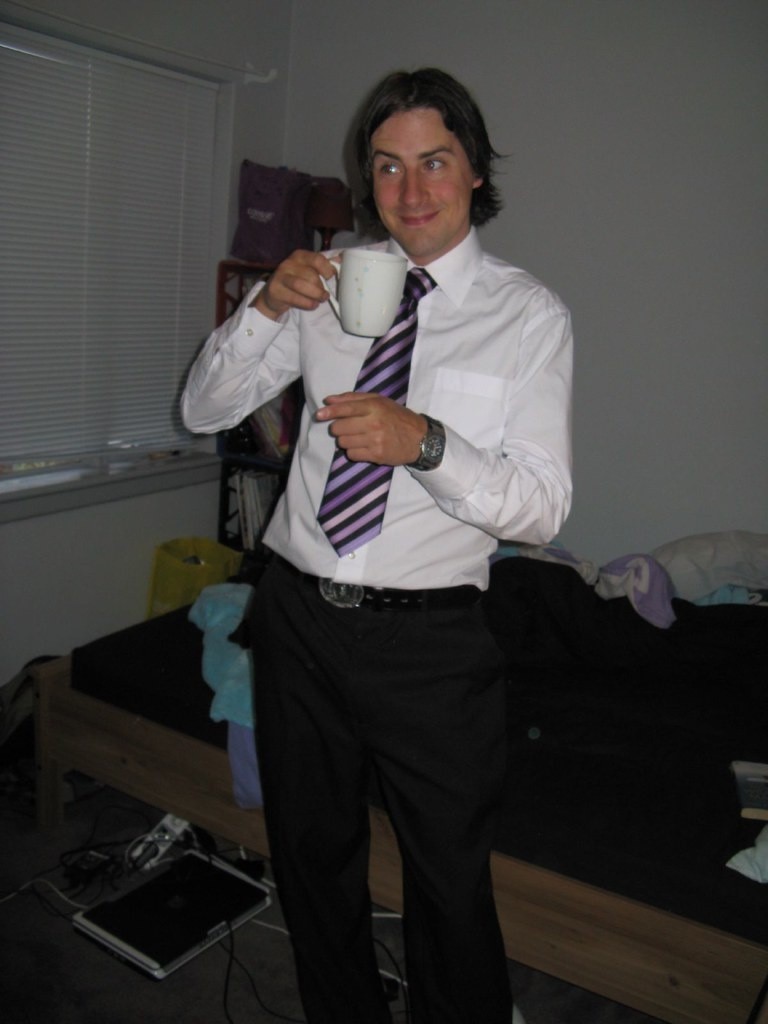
[[72, 848, 271, 980]]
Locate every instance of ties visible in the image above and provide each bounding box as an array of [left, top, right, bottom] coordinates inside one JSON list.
[[316, 267, 437, 559]]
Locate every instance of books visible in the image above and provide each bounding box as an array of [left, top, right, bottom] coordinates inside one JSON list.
[[232, 376, 304, 552], [731, 759, 768, 822]]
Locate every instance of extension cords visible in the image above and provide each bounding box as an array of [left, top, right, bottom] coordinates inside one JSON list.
[[131, 814, 190, 870]]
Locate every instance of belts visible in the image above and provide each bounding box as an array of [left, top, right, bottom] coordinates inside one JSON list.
[[269, 552, 481, 611]]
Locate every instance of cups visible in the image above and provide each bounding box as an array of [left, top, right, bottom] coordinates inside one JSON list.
[[319, 250, 408, 337]]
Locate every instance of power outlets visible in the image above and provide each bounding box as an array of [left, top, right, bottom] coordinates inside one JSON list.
[[131, 813, 190, 870]]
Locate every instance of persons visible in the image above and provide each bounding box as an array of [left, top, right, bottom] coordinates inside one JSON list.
[[179, 64, 576, 1024]]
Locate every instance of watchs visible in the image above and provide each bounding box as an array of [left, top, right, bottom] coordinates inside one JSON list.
[[411, 412, 447, 472]]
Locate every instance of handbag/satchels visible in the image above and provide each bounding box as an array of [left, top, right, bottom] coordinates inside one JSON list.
[[0, 656, 62, 768], [146, 535, 245, 620]]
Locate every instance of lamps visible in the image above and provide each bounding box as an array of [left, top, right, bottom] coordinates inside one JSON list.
[[303, 178, 354, 251]]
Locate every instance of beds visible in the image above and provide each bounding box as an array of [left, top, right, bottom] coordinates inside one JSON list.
[[29, 603, 768, 1024]]
